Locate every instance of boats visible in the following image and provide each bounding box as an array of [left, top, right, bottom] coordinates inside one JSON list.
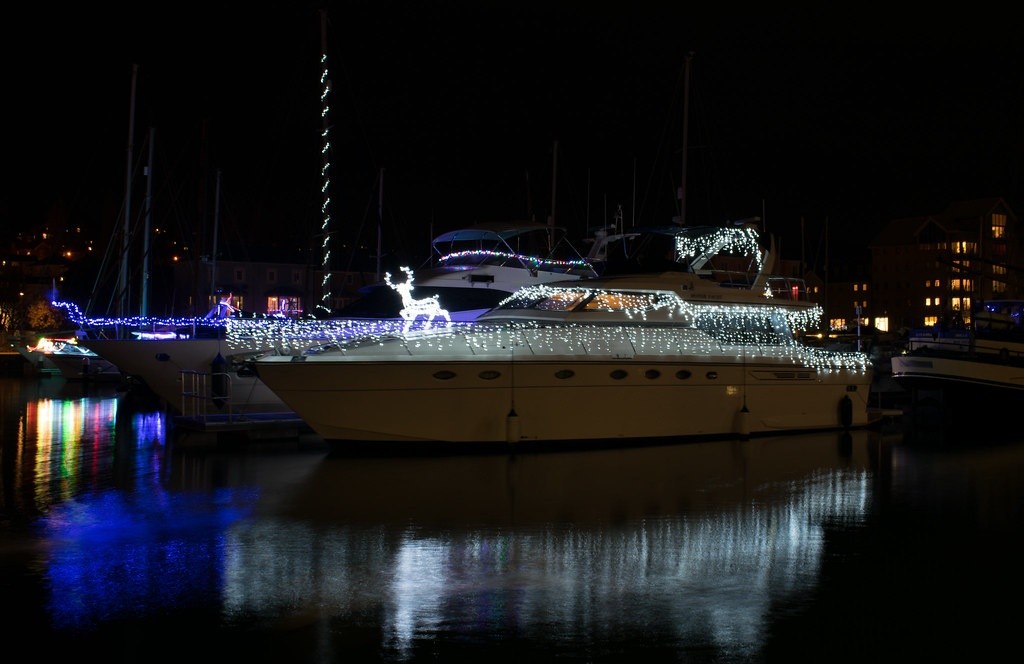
[[74, 57, 904, 445], [890, 216, 1023, 406]]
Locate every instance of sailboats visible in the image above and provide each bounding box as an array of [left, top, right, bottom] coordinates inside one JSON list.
[[16, 62, 157, 376]]
